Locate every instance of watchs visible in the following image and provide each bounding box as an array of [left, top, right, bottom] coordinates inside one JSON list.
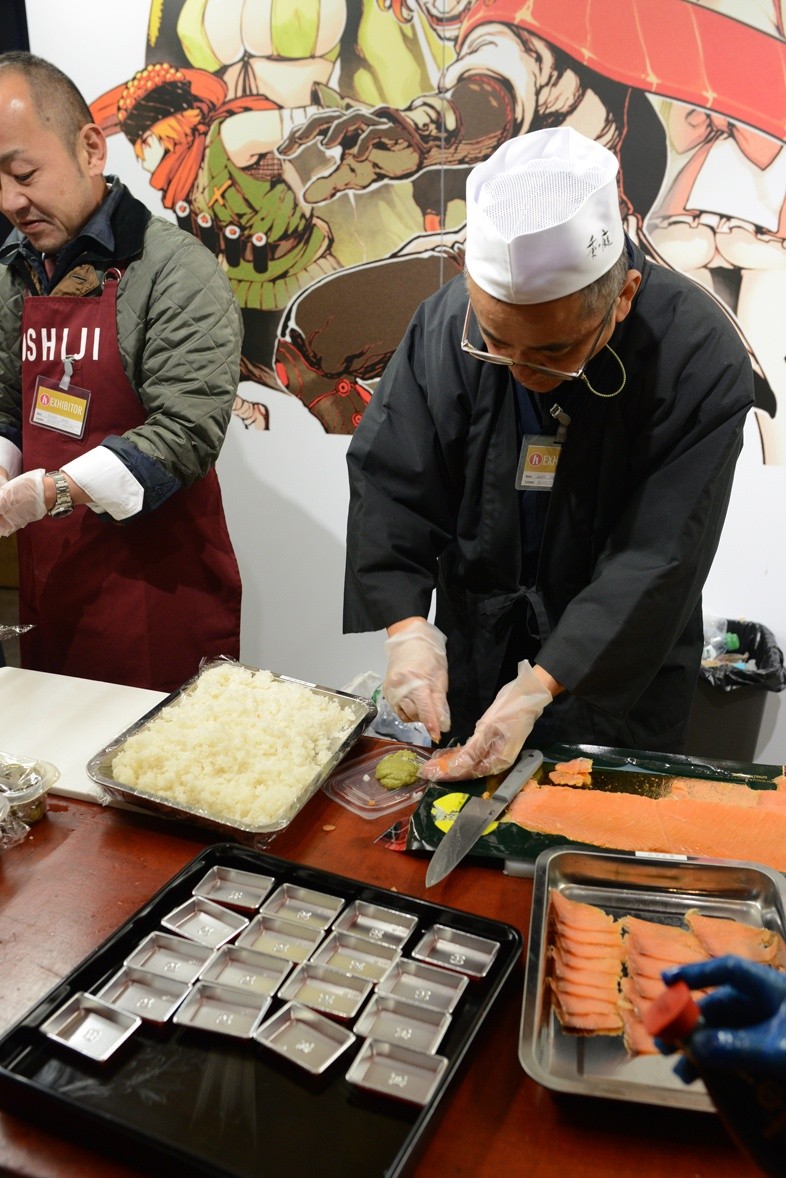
[[45, 470, 74, 518]]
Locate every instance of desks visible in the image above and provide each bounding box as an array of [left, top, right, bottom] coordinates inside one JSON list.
[[0, 736, 786, 1178]]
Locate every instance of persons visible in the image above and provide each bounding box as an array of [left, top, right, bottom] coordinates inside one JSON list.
[[0, 52, 245, 696], [342, 126, 751, 783], [654, 958, 786, 1176]]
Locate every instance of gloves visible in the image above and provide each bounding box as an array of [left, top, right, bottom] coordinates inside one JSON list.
[[654, 956, 786, 1178]]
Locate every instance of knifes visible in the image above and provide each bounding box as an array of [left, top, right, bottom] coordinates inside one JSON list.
[[424, 749, 544, 887]]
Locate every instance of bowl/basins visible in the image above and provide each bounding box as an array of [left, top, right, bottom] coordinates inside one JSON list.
[[0, 750, 62, 824]]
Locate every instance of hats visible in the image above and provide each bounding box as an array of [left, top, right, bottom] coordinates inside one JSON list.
[[463, 126, 626, 306]]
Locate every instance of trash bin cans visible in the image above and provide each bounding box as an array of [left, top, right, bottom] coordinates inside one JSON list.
[[682, 619, 786, 763]]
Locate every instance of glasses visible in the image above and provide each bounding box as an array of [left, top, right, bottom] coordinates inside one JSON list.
[[461, 294, 617, 383]]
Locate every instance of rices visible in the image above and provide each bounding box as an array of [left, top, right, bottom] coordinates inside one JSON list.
[[111, 664, 356, 826]]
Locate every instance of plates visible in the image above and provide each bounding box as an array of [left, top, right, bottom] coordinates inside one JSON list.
[[39, 867, 502, 1107]]
